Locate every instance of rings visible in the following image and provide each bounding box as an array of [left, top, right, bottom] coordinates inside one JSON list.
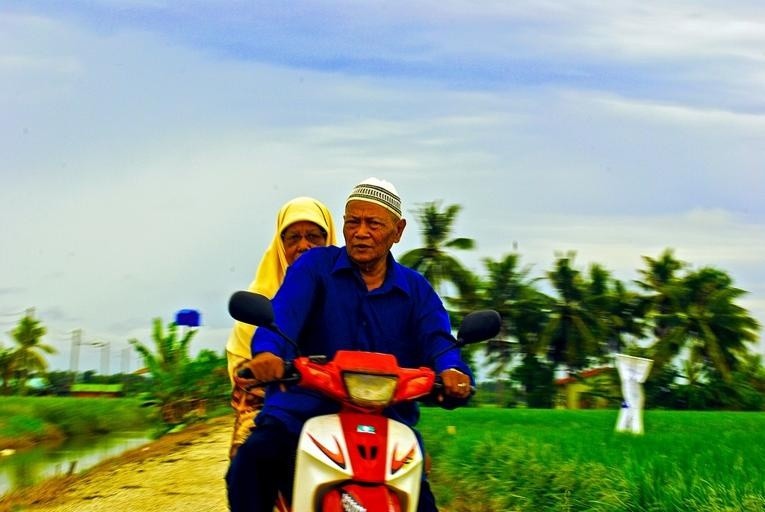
[[458, 383, 466, 387]]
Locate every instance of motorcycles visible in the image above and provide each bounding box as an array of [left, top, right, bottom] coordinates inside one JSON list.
[[228, 290, 502, 512]]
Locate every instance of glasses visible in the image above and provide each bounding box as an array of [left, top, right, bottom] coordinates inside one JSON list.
[[281, 230, 327, 243]]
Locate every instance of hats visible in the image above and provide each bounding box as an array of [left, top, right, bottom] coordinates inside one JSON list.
[[343, 177, 403, 221]]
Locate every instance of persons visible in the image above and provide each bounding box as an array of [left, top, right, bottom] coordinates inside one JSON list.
[[226, 180, 475, 512], [228, 197, 336, 459]]
